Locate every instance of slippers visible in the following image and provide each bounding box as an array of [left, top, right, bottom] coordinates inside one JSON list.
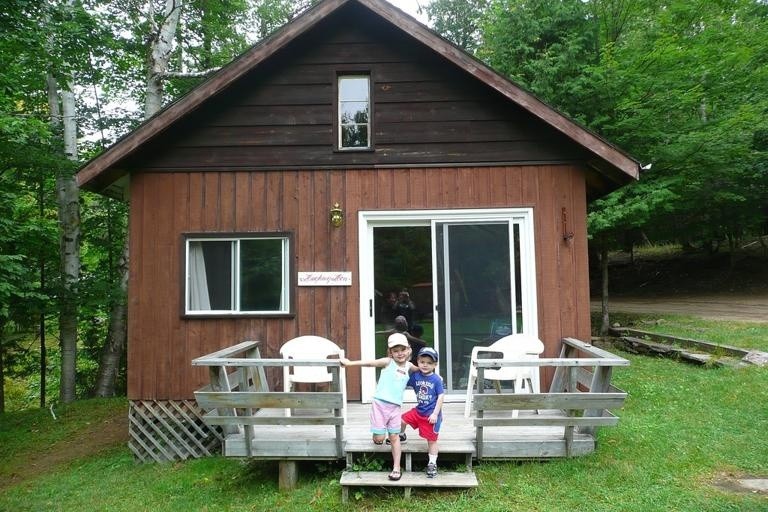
[[388, 471, 402, 481], [373, 436, 383, 445]]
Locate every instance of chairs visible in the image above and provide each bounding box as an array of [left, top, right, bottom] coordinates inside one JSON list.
[[461, 332, 544, 426], [278, 333, 347, 427]]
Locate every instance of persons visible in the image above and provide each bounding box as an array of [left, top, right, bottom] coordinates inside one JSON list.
[[385, 289, 427, 361], [341, 334, 445, 481]]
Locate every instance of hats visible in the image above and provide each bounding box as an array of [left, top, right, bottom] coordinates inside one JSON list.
[[418, 347, 438, 362], [388, 333, 408, 348], [410, 324, 424, 336], [395, 316, 407, 325]]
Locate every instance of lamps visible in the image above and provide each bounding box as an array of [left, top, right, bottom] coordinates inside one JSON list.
[[329, 203, 345, 228]]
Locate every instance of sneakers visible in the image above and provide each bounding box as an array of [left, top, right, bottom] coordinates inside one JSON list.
[[386, 432, 407, 444], [424, 463, 438, 478]]
